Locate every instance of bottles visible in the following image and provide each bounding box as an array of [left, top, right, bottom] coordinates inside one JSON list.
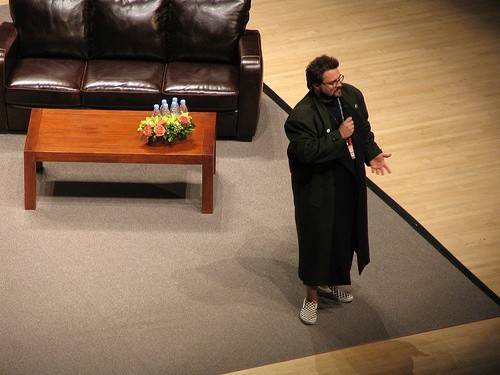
[[152, 97, 188, 117]]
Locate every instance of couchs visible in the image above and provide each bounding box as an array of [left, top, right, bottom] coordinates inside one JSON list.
[[0, 0, 264, 143]]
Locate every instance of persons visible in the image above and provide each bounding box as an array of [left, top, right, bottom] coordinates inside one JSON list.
[[284, 56, 391, 323]]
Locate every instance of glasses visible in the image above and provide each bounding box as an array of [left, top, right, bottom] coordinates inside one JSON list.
[[323, 74, 345, 85]]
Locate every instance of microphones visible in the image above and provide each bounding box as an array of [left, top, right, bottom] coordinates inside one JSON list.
[[344, 113, 355, 160]]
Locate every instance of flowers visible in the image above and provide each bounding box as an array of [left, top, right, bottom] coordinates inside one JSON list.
[[136, 110, 195, 144]]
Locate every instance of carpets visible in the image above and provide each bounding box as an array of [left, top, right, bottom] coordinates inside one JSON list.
[[0, 82, 500, 375]]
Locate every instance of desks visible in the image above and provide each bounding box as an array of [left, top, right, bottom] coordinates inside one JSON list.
[[24, 108, 216, 214]]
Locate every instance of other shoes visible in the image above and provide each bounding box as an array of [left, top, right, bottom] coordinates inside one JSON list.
[[319, 285, 354, 303], [300, 297, 318, 324]]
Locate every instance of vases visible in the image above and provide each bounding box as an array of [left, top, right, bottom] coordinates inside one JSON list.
[[148, 134, 164, 145], [177, 135, 186, 141]]
